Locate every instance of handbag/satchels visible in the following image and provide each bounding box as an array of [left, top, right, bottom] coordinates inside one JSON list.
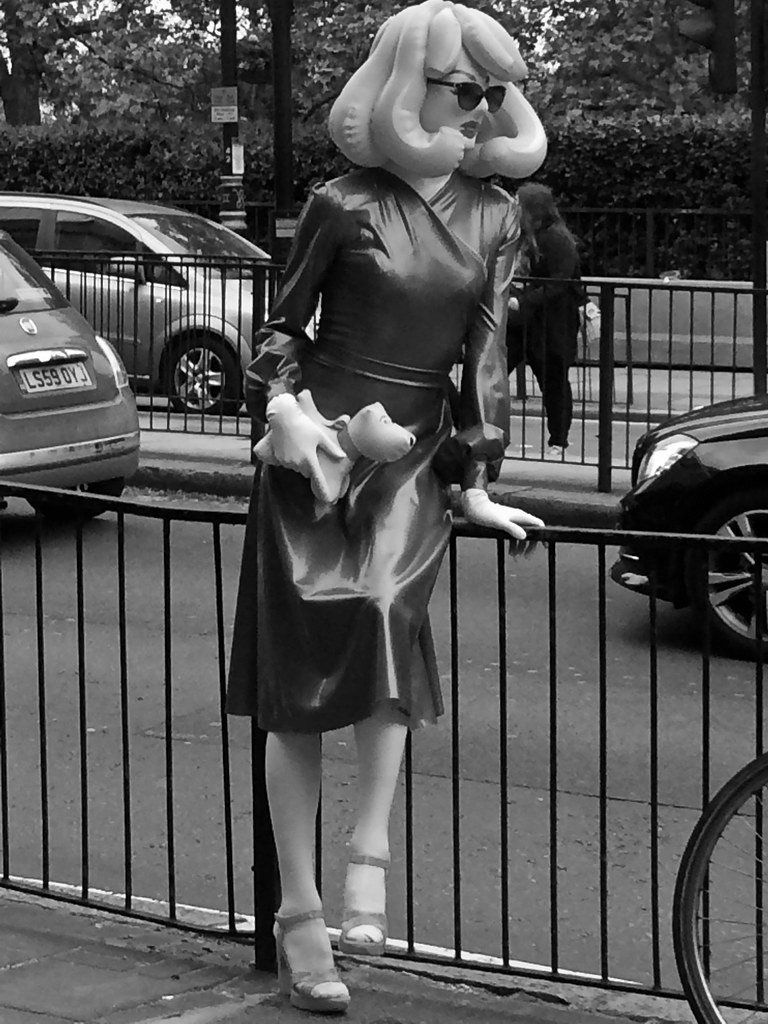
[[578, 301, 601, 347]]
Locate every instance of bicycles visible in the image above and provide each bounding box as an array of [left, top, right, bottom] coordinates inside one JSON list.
[[671, 751, 768, 1024]]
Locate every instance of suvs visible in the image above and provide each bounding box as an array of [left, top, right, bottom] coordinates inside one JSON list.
[[0, 192, 320, 416]]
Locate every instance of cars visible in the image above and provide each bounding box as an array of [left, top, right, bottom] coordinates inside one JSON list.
[[611, 393, 768, 665], [0, 230, 139, 519]]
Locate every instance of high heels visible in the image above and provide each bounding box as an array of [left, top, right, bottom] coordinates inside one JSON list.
[[338, 854, 390, 955], [273, 910, 351, 1011]]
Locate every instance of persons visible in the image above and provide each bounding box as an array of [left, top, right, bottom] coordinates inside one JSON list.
[[506, 182, 580, 456], [227, 3, 544, 1012]]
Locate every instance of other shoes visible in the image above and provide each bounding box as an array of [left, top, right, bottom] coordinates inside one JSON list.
[[543, 445, 566, 461]]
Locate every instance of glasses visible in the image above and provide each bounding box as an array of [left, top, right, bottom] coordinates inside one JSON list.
[[426, 76, 506, 113]]
